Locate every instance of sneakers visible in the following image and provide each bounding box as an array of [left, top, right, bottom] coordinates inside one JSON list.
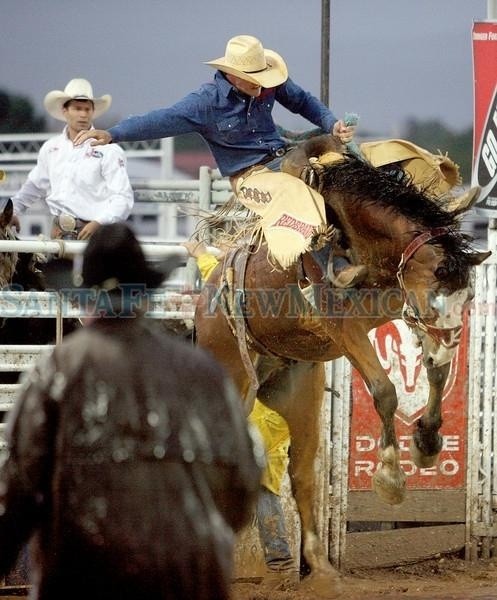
[[332, 264, 369, 300], [439, 186, 481, 214]]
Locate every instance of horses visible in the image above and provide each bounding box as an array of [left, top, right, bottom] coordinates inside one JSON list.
[[161, 151, 492, 585], [0, 196, 186, 423]]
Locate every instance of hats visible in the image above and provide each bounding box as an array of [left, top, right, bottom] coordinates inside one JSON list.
[[44, 78, 112, 122], [42, 222, 180, 306], [199, 35, 289, 89]]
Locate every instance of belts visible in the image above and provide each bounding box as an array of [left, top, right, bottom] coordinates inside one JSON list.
[[230, 144, 292, 177]]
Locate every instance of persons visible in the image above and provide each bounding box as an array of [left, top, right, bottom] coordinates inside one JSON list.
[[12, 79, 135, 240], [74, 35, 481, 289], [181, 241, 297, 575], [0, 224, 260, 600]]
